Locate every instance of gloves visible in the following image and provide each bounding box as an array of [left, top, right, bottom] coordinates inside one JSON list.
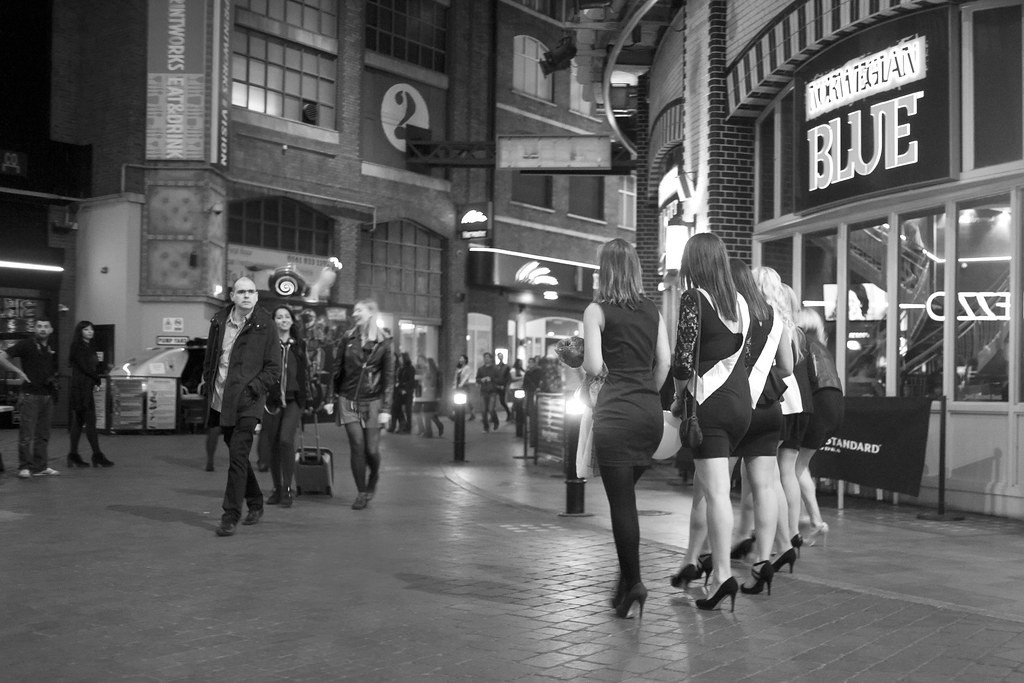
[[670, 393, 684, 418]]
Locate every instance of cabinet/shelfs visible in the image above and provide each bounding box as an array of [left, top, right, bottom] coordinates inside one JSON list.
[[93, 377, 179, 432]]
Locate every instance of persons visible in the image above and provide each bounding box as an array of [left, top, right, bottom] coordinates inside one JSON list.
[[206, 409, 233, 471], [422, 357, 444, 438], [413, 354, 426, 435], [522, 355, 547, 404], [778, 282, 844, 558], [0, 314, 61, 478], [582, 236, 671, 618], [323, 298, 394, 509], [263, 305, 307, 506], [671, 232, 752, 613], [257, 426, 270, 472], [475, 352, 504, 434], [729, 255, 794, 594], [489, 352, 511, 422], [202, 276, 283, 536], [447, 354, 476, 422], [66, 320, 115, 467], [509, 358, 526, 422], [386, 352, 415, 434]]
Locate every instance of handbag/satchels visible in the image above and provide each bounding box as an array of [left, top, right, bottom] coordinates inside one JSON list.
[[679, 414, 703, 450], [575, 407, 600, 478]]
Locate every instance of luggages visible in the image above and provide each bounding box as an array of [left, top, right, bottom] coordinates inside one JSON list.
[[292, 410, 334, 497]]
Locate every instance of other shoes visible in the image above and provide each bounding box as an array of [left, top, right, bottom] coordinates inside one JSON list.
[[267, 489, 281, 505], [352, 494, 369, 509], [256, 460, 269, 473], [280, 487, 294, 508], [385, 413, 512, 440], [367, 475, 378, 499], [205, 465, 214, 471], [242, 507, 265, 525], [18, 447, 115, 477], [215, 519, 237, 534]]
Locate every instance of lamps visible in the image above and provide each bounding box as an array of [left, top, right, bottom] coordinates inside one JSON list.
[[632, 26, 641, 43], [540, 36, 578, 75]]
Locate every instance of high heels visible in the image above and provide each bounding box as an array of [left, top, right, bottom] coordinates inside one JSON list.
[[770, 533, 803, 559], [695, 576, 738, 611], [609, 582, 648, 623], [772, 547, 797, 574], [740, 559, 773, 596], [669, 563, 695, 591], [692, 553, 713, 587], [730, 534, 756, 561], [803, 522, 829, 547]]
